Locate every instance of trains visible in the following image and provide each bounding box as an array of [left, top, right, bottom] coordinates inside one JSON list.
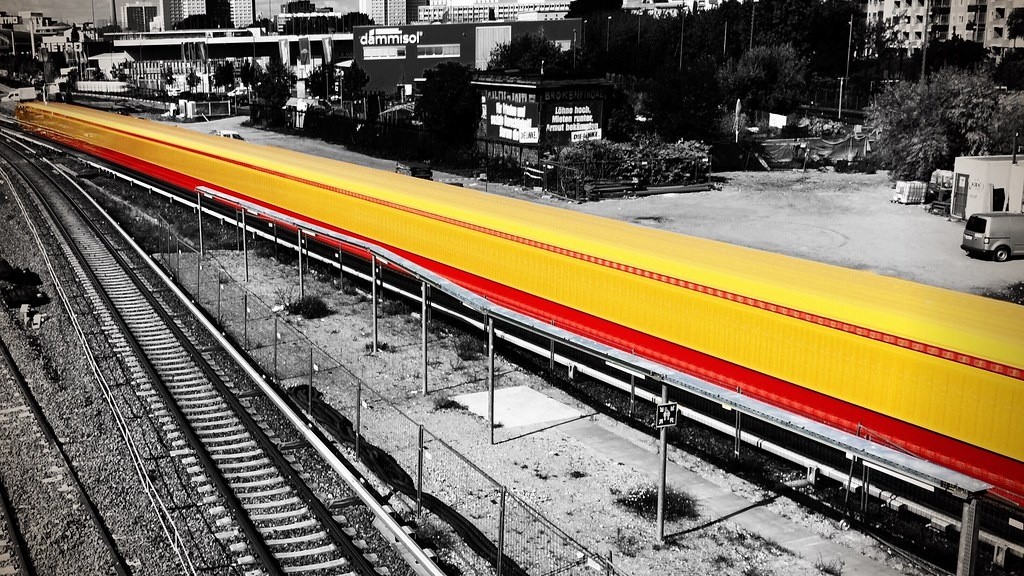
[[14, 101, 1024, 508]]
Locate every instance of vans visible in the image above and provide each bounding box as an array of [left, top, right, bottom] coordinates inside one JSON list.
[[960, 212, 1024, 262]]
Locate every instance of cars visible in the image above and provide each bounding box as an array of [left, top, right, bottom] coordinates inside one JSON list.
[[210, 130, 248, 142]]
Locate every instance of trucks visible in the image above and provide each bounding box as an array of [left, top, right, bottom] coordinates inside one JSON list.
[[0, 86, 37, 103]]
